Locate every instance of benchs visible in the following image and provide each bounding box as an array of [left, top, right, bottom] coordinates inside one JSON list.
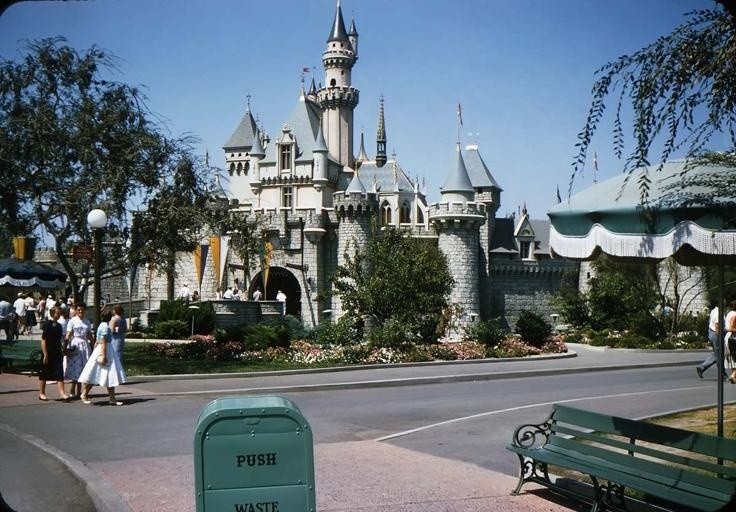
[[0, 338, 45, 378], [507, 404, 736, 512]]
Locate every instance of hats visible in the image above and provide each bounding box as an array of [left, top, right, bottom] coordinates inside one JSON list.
[[18, 292, 23, 298]]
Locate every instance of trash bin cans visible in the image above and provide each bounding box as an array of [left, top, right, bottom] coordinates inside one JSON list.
[[193, 396, 316, 512]]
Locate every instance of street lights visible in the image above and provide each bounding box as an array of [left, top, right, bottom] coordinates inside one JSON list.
[[87, 208, 107, 339]]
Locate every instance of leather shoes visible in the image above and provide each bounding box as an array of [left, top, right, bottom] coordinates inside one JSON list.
[[109, 400, 124, 406], [59, 389, 92, 404], [38, 394, 48, 401]]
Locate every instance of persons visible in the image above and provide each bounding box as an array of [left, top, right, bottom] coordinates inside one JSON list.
[[654, 303, 674, 319], [275, 290, 287, 316], [696, 299, 729, 379], [723, 301, 736, 383], [38, 306, 70, 401], [63, 302, 96, 399], [109, 306, 127, 360], [77, 310, 128, 406], [177, 283, 264, 302], [0, 292, 75, 340]]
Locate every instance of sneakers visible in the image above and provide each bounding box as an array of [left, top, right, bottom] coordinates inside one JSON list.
[[723, 375, 736, 384], [696, 366, 704, 378]]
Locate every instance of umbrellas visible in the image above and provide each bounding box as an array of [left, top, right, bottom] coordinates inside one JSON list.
[[0, 258, 68, 338], [546, 158, 736, 479]]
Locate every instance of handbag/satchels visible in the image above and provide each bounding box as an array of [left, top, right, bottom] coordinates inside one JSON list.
[[96, 356, 110, 367]]
